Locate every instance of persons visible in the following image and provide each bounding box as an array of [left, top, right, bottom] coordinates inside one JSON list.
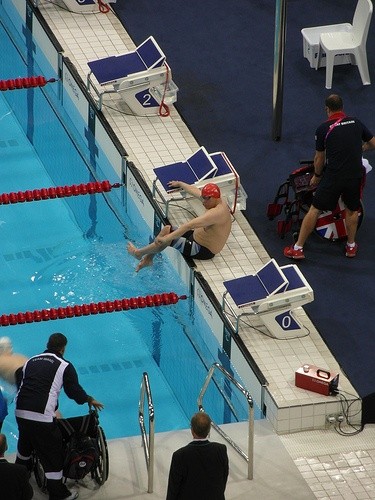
[[14, 332, 105, 500], [0, 433, 34, 500], [0, 336, 61, 419], [125, 180, 231, 272], [0, 389, 8, 432], [281, 94, 375, 260], [165, 413, 229, 500]]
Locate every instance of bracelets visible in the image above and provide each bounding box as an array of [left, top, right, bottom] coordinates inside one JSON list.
[[314, 172, 320, 177]]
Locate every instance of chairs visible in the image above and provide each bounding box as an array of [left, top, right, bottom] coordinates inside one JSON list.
[[315, 0, 373, 89]]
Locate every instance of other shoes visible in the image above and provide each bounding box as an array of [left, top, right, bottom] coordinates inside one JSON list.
[[49, 489, 79, 500]]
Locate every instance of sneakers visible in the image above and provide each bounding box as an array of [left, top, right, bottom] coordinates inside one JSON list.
[[283, 243, 304, 259], [346, 243, 358, 257]]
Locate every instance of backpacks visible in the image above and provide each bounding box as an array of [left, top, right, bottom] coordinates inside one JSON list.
[[58, 415, 100, 480]]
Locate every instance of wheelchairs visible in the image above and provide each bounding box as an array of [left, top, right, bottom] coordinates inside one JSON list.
[[266, 144, 371, 244], [32, 404, 110, 489]]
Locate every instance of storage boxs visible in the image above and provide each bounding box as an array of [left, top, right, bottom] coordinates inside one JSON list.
[[301, 23, 353, 69]]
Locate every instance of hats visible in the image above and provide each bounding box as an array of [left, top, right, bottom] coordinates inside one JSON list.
[[202, 184, 220, 197]]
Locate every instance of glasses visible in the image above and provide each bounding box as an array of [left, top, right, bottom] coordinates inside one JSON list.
[[200, 197, 211, 201]]
